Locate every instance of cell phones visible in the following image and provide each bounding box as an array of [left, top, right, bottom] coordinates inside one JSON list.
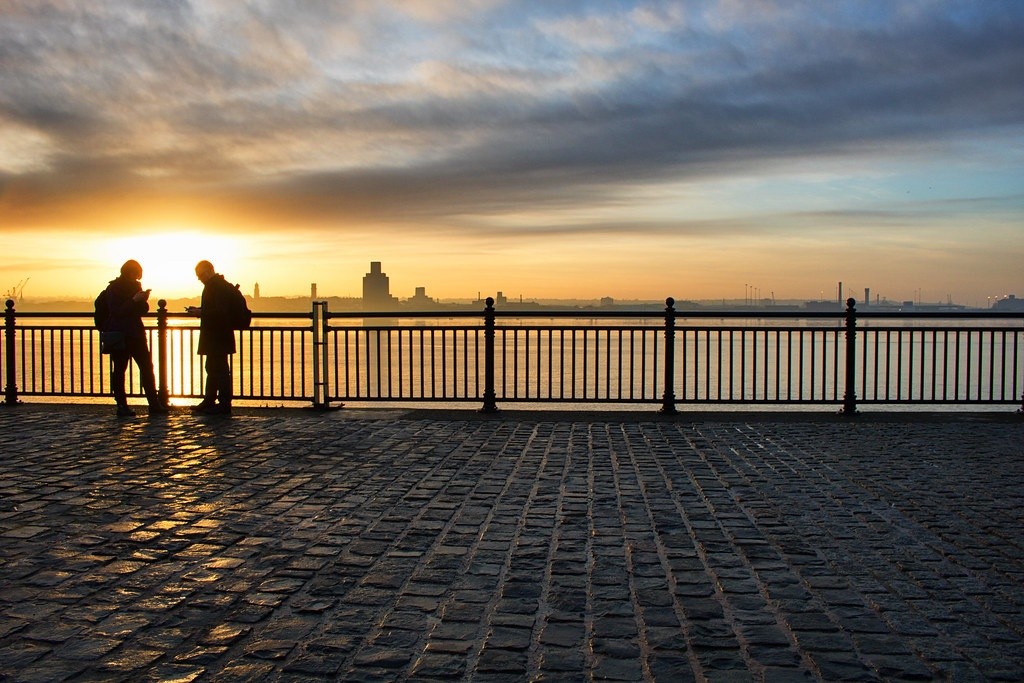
[[145, 289, 151, 293], [184, 307, 189, 310]]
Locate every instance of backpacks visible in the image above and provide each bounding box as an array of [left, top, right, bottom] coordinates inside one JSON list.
[[94, 281, 125, 332], [207, 281, 251, 330]]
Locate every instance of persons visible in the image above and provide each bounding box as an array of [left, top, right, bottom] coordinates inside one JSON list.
[[187, 260, 237, 415], [101, 260, 170, 417]]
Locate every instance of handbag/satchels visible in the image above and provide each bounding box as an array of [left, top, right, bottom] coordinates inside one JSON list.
[[100, 330, 126, 354]]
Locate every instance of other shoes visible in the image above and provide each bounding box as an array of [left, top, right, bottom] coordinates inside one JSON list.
[[116, 405, 137, 416], [189, 398, 231, 416], [149, 400, 169, 413]]
